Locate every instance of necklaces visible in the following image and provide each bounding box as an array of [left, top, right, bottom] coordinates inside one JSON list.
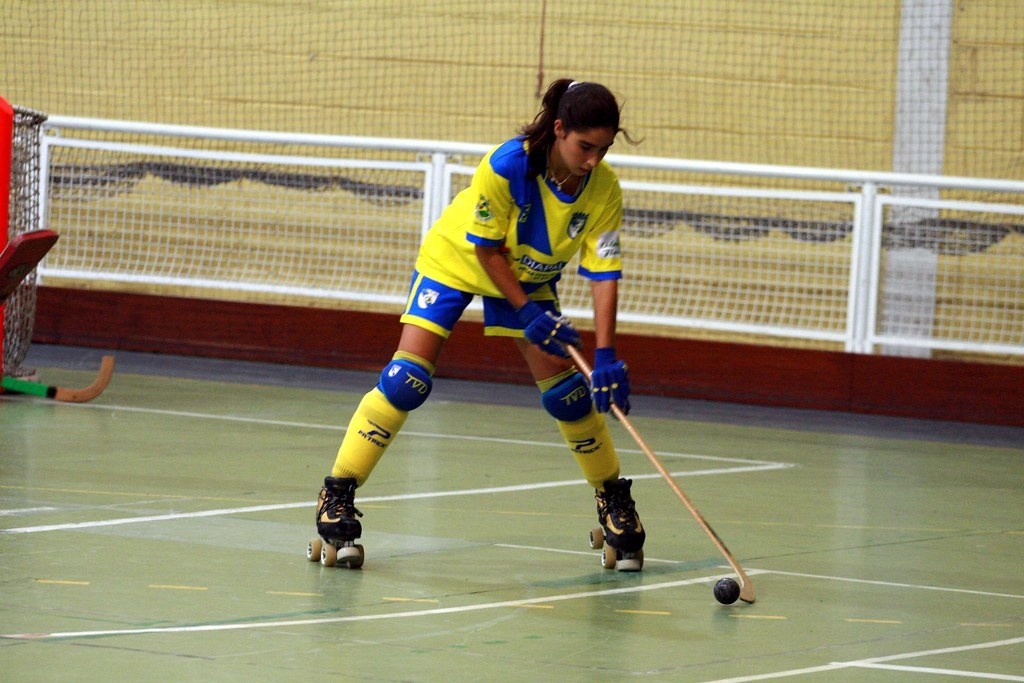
[[550, 167, 572, 191]]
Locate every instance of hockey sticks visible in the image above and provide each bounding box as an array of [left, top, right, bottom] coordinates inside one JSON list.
[[544, 305, 757, 605], [1, 354, 115, 403]]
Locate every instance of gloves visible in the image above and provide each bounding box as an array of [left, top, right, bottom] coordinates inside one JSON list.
[[589, 346, 630, 412], [515, 300, 579, 356]]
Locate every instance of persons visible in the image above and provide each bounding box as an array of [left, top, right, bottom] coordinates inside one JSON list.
[[307, 79, 646, 573]]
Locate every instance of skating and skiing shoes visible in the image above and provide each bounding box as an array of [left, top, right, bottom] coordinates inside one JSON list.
[[589, 477, 645, 571], [307, 476, 364, 569]]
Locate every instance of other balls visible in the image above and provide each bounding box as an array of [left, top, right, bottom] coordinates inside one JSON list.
[[714, 577, 741, 606]]
